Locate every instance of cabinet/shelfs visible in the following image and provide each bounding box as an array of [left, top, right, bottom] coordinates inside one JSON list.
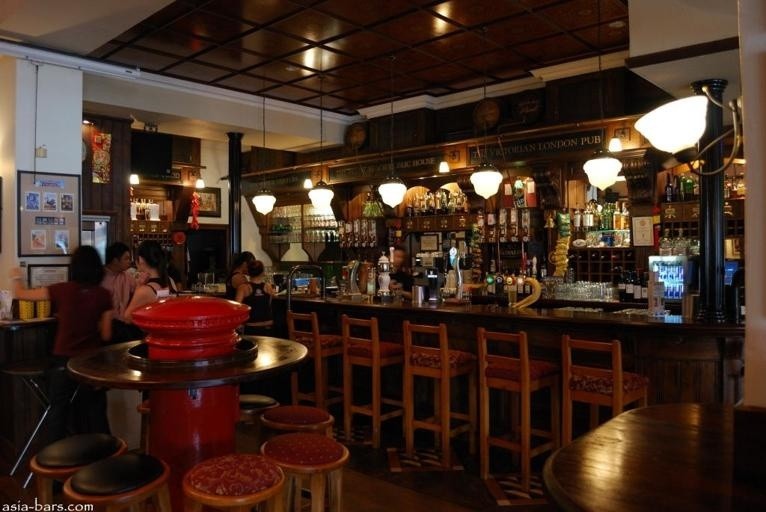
[[80, 112, 745, 375]]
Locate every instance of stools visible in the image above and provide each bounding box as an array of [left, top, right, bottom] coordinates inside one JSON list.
[[0, 361, 351, 511]]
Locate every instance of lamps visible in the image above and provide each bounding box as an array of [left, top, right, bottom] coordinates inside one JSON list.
[[580, 1, 624, 192], [374, 1, 408, 210], [469, 1, 506, 203], [251, 59, 278, 217], [307, 1, 335, 213], [633, 82, 745, 175]]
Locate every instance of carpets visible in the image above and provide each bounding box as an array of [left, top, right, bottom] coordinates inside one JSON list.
[[235, 400, 565, 509]]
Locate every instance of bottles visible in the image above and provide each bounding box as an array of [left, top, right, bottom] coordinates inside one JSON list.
[[646, 270, 664, 319], [407, 189, 470, 217], [473, 243, 547, 295], [568, 200, 633, 261], [265, 205, 339, 242], [541, 276, 613, 303], [266, 271, 314, 294], [340, 262, 377, 297], [614, 261, 688, 302], [654, 227, 699, 256], [663, 171, 745, 201]]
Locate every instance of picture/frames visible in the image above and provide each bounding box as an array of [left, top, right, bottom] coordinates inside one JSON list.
[[27, 263, 70, 288], [16, 169, 81, 256]]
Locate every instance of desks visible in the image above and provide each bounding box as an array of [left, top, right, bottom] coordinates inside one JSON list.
[[543, 399, 766, 510], [66, 335, 310, 509]]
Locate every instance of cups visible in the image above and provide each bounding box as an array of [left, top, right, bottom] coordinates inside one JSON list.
[[412, 284, 425, 304], [681, 293, 701, 319], [204, 273, 215, 292]]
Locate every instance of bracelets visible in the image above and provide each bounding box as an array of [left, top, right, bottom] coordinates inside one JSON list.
[[11, 276, 24, 283]]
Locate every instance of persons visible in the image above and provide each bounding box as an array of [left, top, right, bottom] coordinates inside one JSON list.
[[123, 240, 177, 340], [98, 242, 145, 344], [163, 248, 183, 291], [234, 260, 273, 336], [225, 250, 256, 301], [9, 245, 111, 444]]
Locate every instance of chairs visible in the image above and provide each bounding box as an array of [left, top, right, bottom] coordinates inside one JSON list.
[[284, 308, 651, 492]]
